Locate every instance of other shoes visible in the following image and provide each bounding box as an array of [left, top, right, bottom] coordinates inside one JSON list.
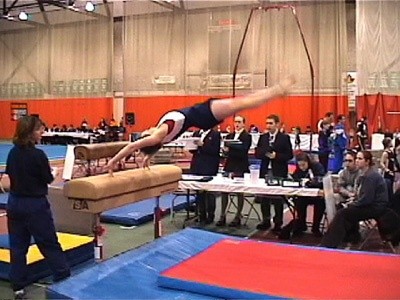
[[284, 219, 308, 234], [311, 226, 324, 238], [256, 218, 271, 230], [228, 217, 242, 228], [199, 214, 215, 227], [269, 223, 283, 233], [13, 288, 34, 300], [52, 269, 71, 283], [216, 215, 227, 227], [342, 230, 361, 244]]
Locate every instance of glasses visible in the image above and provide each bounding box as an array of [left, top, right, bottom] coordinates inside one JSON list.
[[344, 159, 353, 163]]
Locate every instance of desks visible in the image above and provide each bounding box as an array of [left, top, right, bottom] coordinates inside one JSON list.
[[41, 131, 94, 143], [163, 145, 186, 158], [163, 133, 385, 151], [178, 174, 324, 244]]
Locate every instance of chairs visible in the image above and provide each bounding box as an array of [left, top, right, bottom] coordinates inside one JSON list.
[[171, 191, 197, 219], [227, 193, 260, 226], [358, 188, 400, 252]]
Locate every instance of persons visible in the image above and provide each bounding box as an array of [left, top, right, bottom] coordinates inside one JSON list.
[[381, 128, 400, 189], [317, 112, 350, 174], [41, 117, 123, 145], [250, 124, 258, 132], [216, 114, 252, 226], [357, 116, 369, 150], [217, 125, 231, 133], [104, 75, 296, 177], [294, 153, 327, 235], [2, 113, 71, 300], [279, 123, 313, 133], [254, 114, 293, 232], [189, 127, 220, 225], [320, 150, 400, 247]]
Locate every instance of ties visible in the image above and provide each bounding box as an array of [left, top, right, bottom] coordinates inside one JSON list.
[[268, 136, 274, 147]]
